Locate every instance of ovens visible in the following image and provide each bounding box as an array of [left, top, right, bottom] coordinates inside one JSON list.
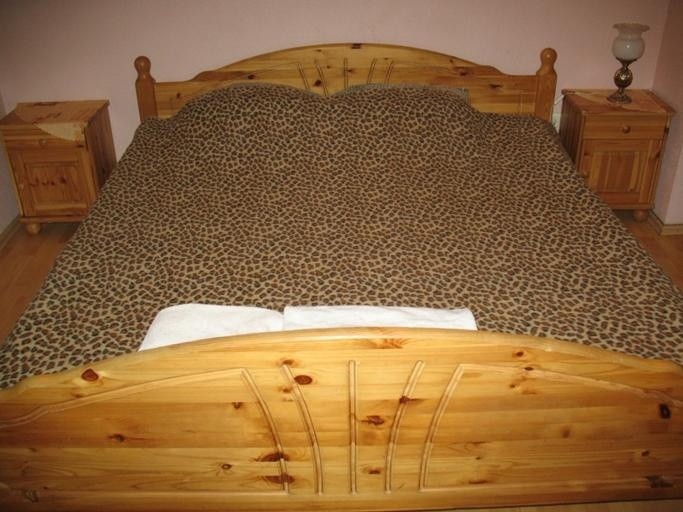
[[0, 43, 683, 512]]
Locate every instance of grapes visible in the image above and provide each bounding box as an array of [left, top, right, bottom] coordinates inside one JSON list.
[[561, 90, 676, 223], [0, 99, 114, 234]]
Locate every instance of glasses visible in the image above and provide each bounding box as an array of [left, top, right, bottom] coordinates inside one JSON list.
[[607, 24, 650, 104]]
